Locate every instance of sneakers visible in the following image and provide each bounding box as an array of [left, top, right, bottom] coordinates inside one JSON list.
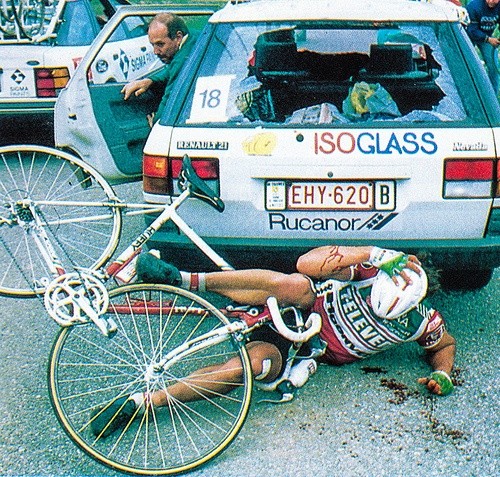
[[137, 249, 184, 290], [90, 393, 138, 438]]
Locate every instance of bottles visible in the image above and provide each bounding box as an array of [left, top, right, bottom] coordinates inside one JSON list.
[[288, 357, 317, 387]]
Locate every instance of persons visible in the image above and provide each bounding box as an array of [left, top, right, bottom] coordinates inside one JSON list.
[[89, 244, 455, 437], [121, 11, 202, 128], [462, 0, 500, 105]]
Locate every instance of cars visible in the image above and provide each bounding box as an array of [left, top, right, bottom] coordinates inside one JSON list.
[[0, 0, 148, 140], [51, 1, 500, 296]]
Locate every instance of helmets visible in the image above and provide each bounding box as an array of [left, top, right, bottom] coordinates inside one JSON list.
[[368, 259, 430, 320]]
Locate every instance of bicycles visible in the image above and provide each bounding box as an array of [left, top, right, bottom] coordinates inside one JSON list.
[[0, 142, 322, 476]]
